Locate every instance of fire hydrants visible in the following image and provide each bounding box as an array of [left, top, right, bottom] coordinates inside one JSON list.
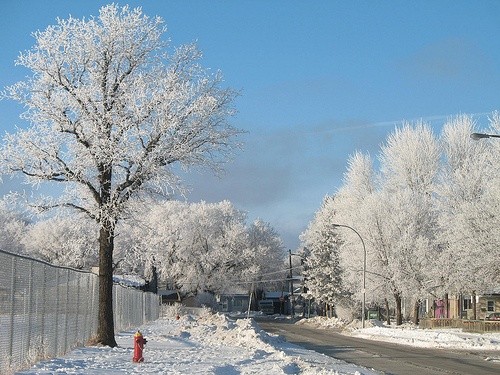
[[133, 331, 148, 363]]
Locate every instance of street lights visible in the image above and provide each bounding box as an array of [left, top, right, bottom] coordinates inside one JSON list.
[[331, 223, 366, 328], [470, 133, 499, 140], [291, 254, 307, 319]]
